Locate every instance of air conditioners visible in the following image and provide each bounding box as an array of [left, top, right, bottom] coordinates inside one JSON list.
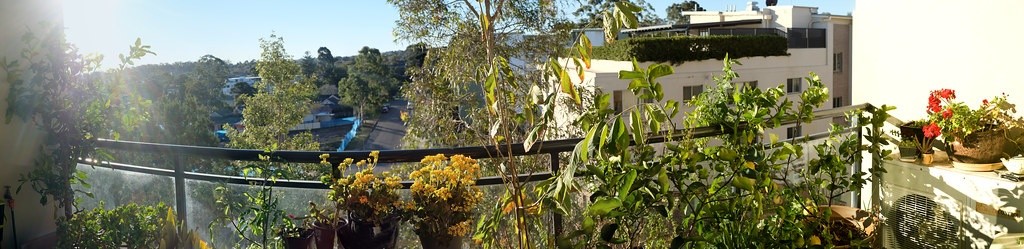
[[878, 150, 1024, 249]]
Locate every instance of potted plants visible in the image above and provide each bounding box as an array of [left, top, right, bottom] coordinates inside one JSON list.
[[900, 120, 931, 139], [282, 200, 337, 249], [665, 56, 895, 249], [898, 137, 916, 159], [914, 134, 935, 164]]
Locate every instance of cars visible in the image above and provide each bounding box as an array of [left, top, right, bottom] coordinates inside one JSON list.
[[381, 107, 389, 113]]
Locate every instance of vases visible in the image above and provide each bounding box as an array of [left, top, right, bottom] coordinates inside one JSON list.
[[338, 221, 400, 249], [951, 127, 1011, 172], [418, 231, 465, 249]]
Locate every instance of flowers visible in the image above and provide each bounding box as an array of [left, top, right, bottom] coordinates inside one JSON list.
[[317, 150, 417, 226], [921, 89, 1024, 152], [399, 153, 487, 237]]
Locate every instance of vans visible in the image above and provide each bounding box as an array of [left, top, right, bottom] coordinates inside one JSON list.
[[407, 101, 414, 110]]
